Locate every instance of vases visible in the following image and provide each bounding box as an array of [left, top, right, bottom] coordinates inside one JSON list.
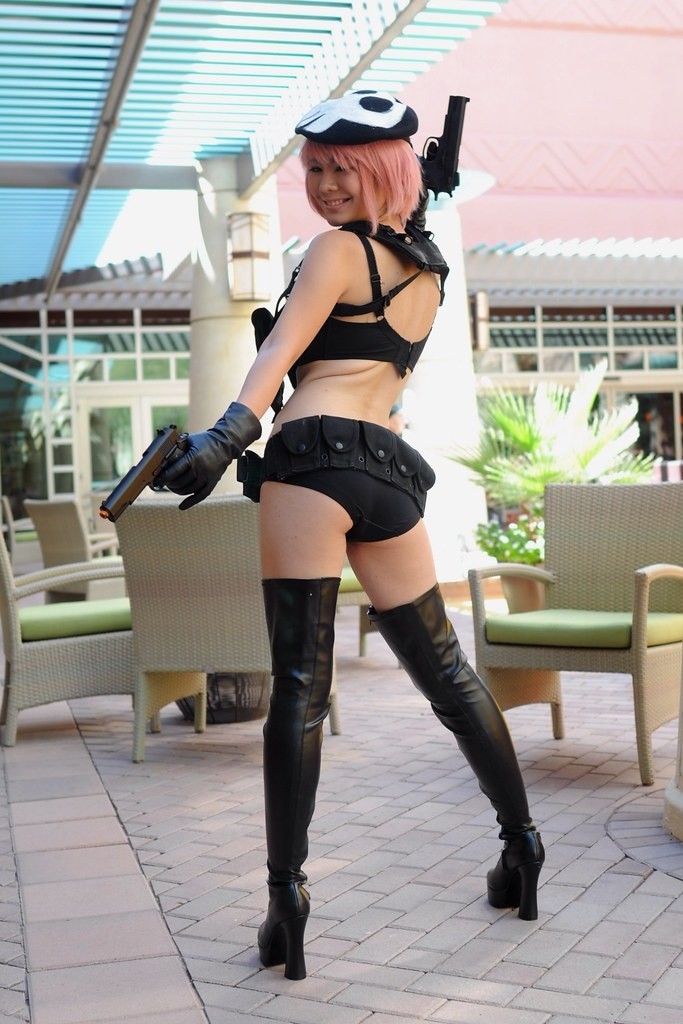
[[497, 561, 550, 613]]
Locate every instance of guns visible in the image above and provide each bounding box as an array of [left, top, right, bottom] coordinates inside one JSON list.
[[416, 95, 471, 201], [98, 424, 192, 524]]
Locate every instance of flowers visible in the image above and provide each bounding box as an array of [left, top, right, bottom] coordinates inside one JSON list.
[[442, 355, 659, 562]]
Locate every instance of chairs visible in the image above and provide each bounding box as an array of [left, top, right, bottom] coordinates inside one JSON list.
[[113, 502, 341, 763], [0, 530, 163, 747], [468, 482, 683, 784], [24, 499, 127, 603]]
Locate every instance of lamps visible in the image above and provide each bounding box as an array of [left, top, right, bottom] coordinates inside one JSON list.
[[224, 211, 275, 303]]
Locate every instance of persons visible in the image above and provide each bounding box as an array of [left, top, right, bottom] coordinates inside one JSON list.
[[144, 88, 548, 983]]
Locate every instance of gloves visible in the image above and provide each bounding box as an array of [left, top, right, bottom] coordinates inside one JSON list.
[[153, 401, 263, 511], [410, 153, 428, 232]]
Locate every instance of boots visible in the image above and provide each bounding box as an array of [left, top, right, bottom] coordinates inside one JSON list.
[[368, 583, 545, 921], [257, 577, 342, 980]]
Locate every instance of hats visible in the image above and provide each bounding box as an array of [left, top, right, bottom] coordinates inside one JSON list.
[[295, 88, 419, 145]]
